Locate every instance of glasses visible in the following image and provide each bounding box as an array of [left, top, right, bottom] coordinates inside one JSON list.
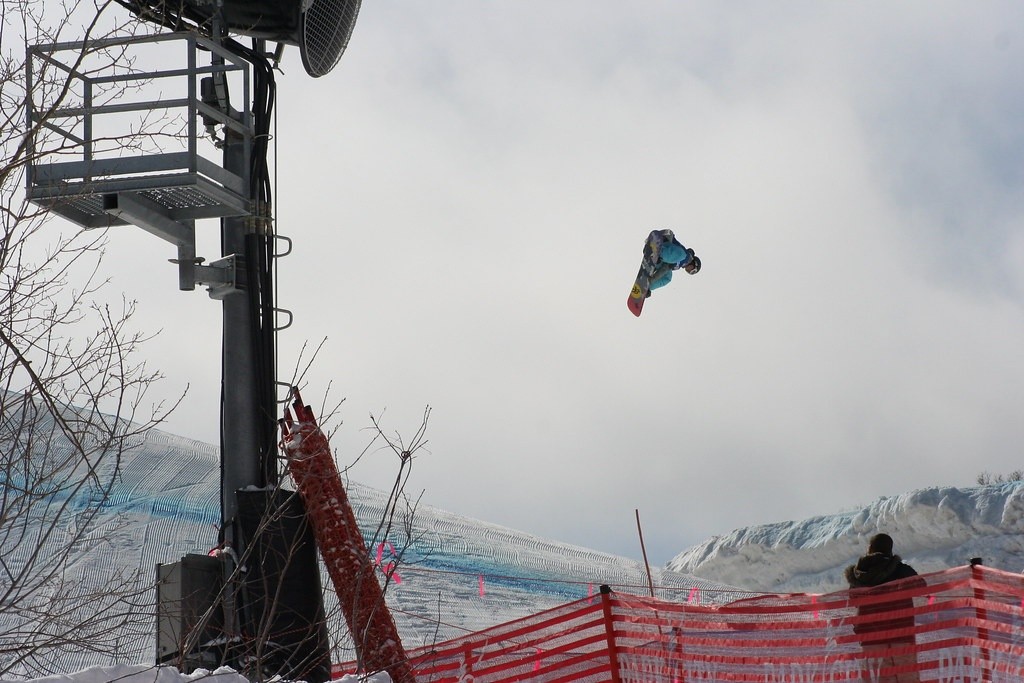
[[685, 270, 697, 275]]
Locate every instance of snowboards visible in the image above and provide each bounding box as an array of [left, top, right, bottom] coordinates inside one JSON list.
[[626, 229, 664, 318]]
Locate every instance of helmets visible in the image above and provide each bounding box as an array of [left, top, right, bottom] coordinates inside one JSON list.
[[685, 255, 701, 277]]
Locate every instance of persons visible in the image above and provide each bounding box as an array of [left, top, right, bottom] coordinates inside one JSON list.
[[844, 534, 928, 683], [643, 229, 701, 299]]
